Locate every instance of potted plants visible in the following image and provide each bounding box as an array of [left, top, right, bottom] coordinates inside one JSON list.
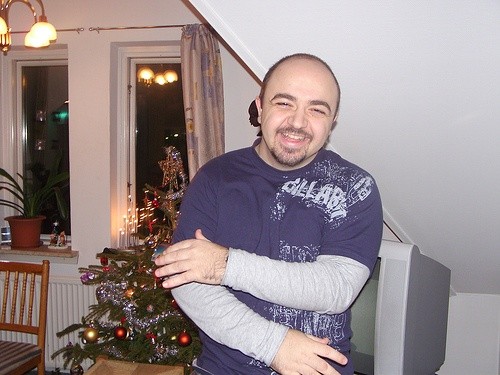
[[0, 158, 71, 248]]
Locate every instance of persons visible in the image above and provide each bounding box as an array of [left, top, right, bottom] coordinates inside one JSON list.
[[154, 53, 384, 375]]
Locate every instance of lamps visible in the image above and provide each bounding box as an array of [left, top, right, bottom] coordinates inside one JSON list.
[[136, 63, 178, 87], [0, 0, 57, 56]]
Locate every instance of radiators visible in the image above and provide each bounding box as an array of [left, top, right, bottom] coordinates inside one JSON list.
[[0, 271, 138, 375]]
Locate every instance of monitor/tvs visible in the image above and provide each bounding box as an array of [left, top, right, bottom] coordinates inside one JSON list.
[[349, 239, 452, 375]]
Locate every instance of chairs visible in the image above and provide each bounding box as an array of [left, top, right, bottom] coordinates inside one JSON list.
[[0, 260, 50, 375]]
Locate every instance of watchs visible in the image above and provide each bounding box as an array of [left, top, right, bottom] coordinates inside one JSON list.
[[225, 254, 228, 263]]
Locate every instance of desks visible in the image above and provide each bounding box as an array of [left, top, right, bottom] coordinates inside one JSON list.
[[83, 355, 186, 375]]
